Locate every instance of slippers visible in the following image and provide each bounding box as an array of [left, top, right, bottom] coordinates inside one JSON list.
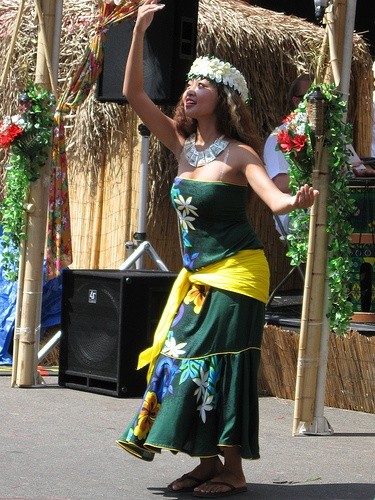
[[167, 473, 204, 496], [193, 480, 248, 497]]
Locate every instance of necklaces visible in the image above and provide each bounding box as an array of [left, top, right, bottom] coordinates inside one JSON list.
[[182, 131, 231, 170]]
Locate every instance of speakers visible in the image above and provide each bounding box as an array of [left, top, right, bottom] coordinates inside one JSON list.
[[57, 265, 181, 399], [95, 0, 198, 105]]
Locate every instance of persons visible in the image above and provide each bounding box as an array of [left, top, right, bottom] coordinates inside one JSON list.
[[262, 73, 374, 311], [114, 1, 319, 500]]
